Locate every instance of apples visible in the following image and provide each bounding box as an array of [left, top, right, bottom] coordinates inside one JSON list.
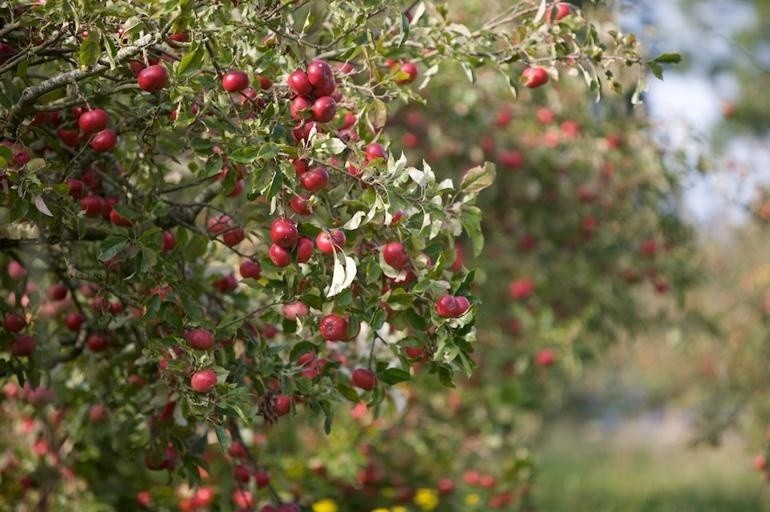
[[0, 0, 769, 512]]
